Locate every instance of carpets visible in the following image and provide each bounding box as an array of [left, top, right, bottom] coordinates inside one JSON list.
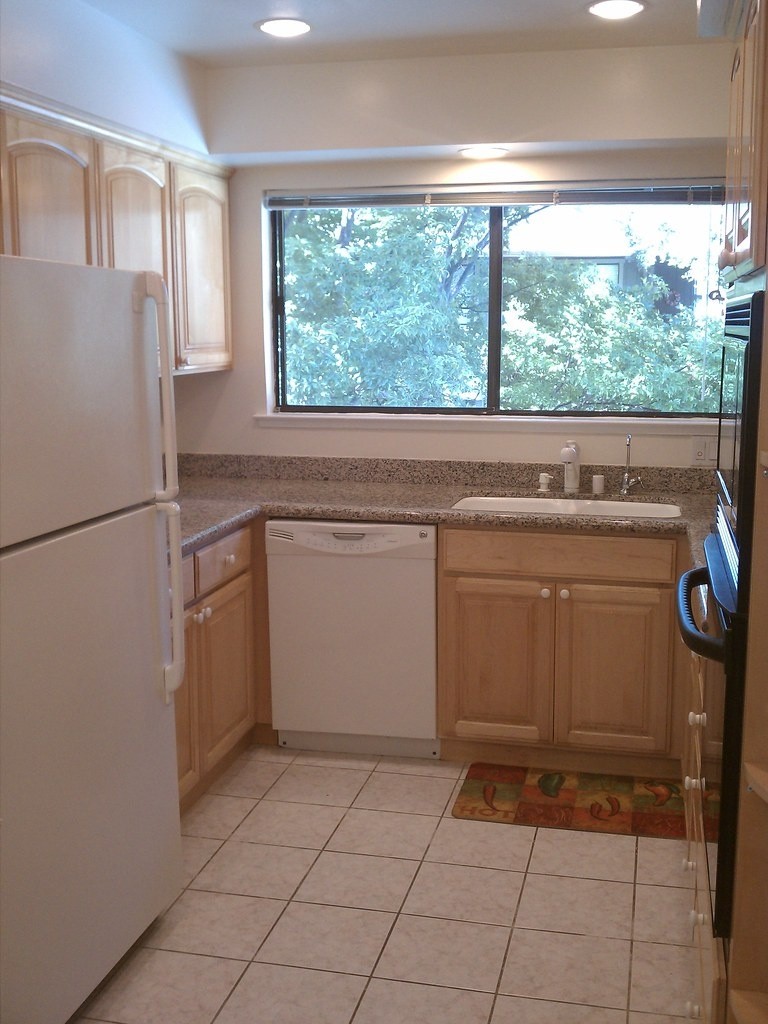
[[453, 761, 688, 840]]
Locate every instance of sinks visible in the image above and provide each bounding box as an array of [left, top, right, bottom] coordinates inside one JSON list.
[[449, 497, 683, 518]]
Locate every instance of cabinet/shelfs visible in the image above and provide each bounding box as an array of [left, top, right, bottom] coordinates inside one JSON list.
[[0, 106, 236, 375], [432, 0, 768, 1024], [178, 527, 256, 814]]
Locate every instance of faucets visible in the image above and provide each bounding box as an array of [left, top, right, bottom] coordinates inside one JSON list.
[[619, 433, 646, 495], [560, 439, 581, 491]]
